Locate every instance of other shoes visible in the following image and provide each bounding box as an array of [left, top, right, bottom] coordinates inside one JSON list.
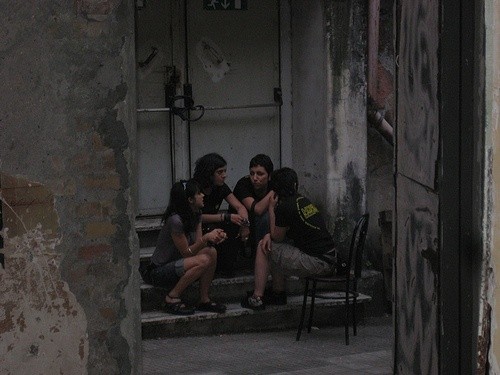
[[216, 271, 236, 279]]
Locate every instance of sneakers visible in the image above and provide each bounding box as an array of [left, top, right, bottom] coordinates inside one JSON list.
[[164, 296, 194, 315], [241, 296, 265, 311], [195, 301, 226, 312]]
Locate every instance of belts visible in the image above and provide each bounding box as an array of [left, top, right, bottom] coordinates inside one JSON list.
[[318, 250, 339, 266], [202, 227, 214, 231]]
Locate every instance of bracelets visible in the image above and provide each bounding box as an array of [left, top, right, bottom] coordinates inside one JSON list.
[[220, 213, 231, 221]]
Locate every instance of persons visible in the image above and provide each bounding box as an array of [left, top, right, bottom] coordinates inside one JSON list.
[[142, 179, 227, 315], [241, 167, 337, 311], [191, 152, 249, 278], [228, 154, 273, 252]]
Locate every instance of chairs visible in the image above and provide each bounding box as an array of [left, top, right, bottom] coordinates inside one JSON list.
[[296, 212, 369, 345]]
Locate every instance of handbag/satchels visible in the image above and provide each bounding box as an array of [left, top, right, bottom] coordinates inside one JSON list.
[[138, 257, 152, 284]]
